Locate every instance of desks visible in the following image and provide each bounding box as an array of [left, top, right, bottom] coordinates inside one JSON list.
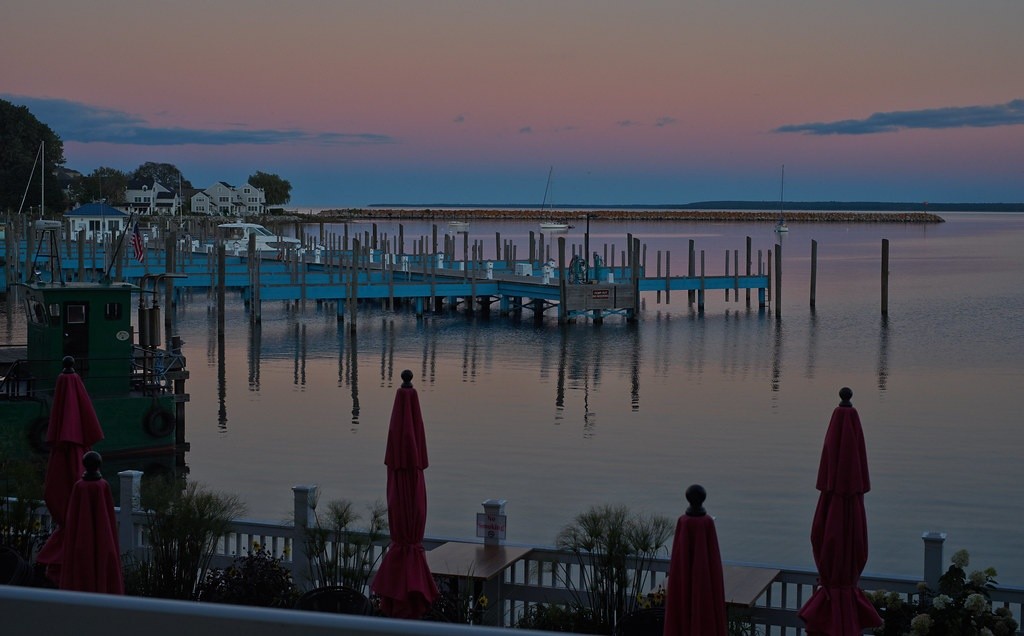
[[425, 541, 533, 629], [647, 564, 781, 634]]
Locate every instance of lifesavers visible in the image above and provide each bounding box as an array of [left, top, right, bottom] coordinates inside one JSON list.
[[145, 406, 176, 439], [30, 417, 52, 452]]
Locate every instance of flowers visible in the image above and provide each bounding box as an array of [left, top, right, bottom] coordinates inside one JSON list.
[[635, 581, 665, 611], [427, 562, 508, 626], [0, 486, 51, 563], [867, 550, 1021, 636], [193, 540, 297, 608]]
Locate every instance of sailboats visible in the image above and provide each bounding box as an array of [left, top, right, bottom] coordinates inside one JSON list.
[[11, 141, 62, 231], [539, 165, 569, 229], [770, 163, 789, 232]]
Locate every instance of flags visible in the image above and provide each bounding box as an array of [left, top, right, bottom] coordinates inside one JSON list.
[[130, 222, 143, 262]]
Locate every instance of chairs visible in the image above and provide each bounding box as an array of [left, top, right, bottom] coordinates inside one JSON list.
[[293, 585, 374, 616], [616, 607, 664, 636]]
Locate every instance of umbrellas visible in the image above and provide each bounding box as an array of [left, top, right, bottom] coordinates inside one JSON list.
[[371, 371, 440, 619], [44, 357, 104, 584], [796, 387, 884, 636], [60, 451, 125, 595], [661, 484, 728, 636]]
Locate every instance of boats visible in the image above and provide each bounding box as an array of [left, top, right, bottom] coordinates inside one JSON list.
[[0, 212, 189, 529], [182, 216, 329, 261], [448, 220, 471, 227]]
